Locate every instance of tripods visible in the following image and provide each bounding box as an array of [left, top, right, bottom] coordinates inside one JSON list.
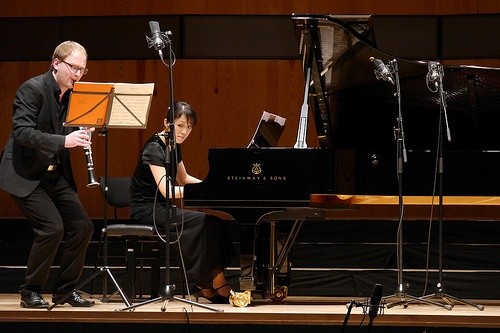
[[46, 91, 154, 310], [116, 43, 224, 313], [346, 60, 452, 312], [385, 83, 486, 312]]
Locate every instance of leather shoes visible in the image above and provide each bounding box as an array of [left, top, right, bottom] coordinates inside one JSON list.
[[19, 290, 49, 309], [52, 290, 96, 307]]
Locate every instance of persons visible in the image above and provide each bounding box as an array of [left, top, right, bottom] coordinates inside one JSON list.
[[0, 39, 98, 309], [126, 100, 236, 303]]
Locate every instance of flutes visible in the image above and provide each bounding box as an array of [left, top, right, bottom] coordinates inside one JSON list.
[[73, 80, 100, 188]]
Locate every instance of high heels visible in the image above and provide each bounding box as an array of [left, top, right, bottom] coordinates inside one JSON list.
[[216, 284, 242, 301], [196, 288, 229, 304]]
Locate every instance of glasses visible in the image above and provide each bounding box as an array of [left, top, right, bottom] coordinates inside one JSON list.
[[62, 59, 89, 75]]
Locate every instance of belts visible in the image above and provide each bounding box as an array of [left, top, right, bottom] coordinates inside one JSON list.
[[47, 163, 61, 172]]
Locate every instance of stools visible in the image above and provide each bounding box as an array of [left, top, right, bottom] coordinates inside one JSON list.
[[103, 223, 159, 300]]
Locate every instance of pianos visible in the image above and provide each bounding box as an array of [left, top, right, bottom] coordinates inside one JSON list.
[[183, 148, 334, 300]]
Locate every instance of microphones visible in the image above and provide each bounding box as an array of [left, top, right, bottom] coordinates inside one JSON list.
[[428, 61, 439, 87], [149, 20, 164, 57], [373, 59, 394, 84]]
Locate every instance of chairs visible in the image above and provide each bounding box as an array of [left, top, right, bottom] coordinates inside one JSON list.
[[99, 176, 163, 301]]
